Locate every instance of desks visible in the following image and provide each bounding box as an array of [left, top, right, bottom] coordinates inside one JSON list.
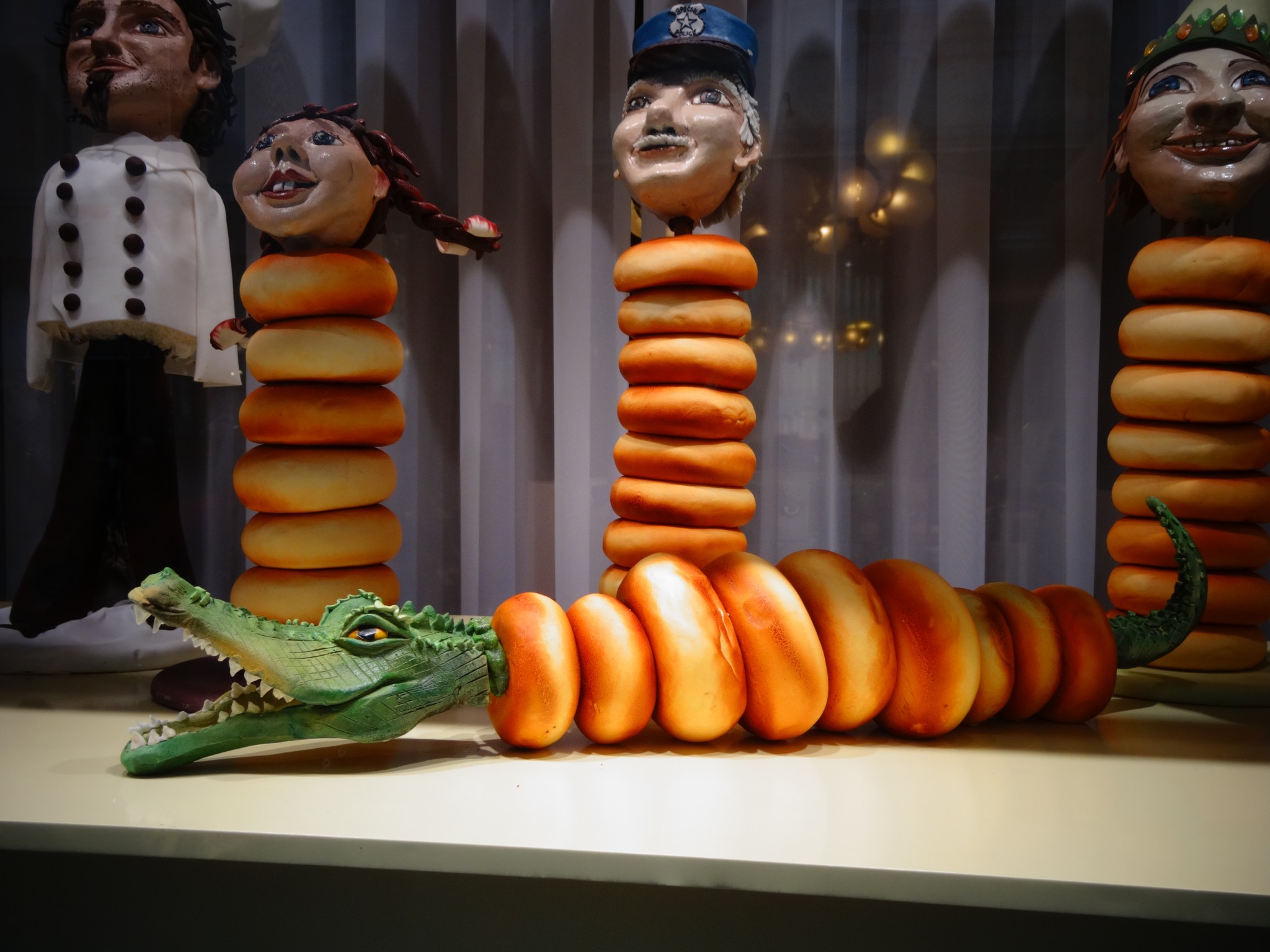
[[1, 669, 1269, 950]]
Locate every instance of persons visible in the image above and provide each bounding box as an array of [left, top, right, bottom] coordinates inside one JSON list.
[[1100, 0, 1270, 222], [234, 102, 501, 262], [610, 4, 764, 226], [8, 0, 239, 638]]
